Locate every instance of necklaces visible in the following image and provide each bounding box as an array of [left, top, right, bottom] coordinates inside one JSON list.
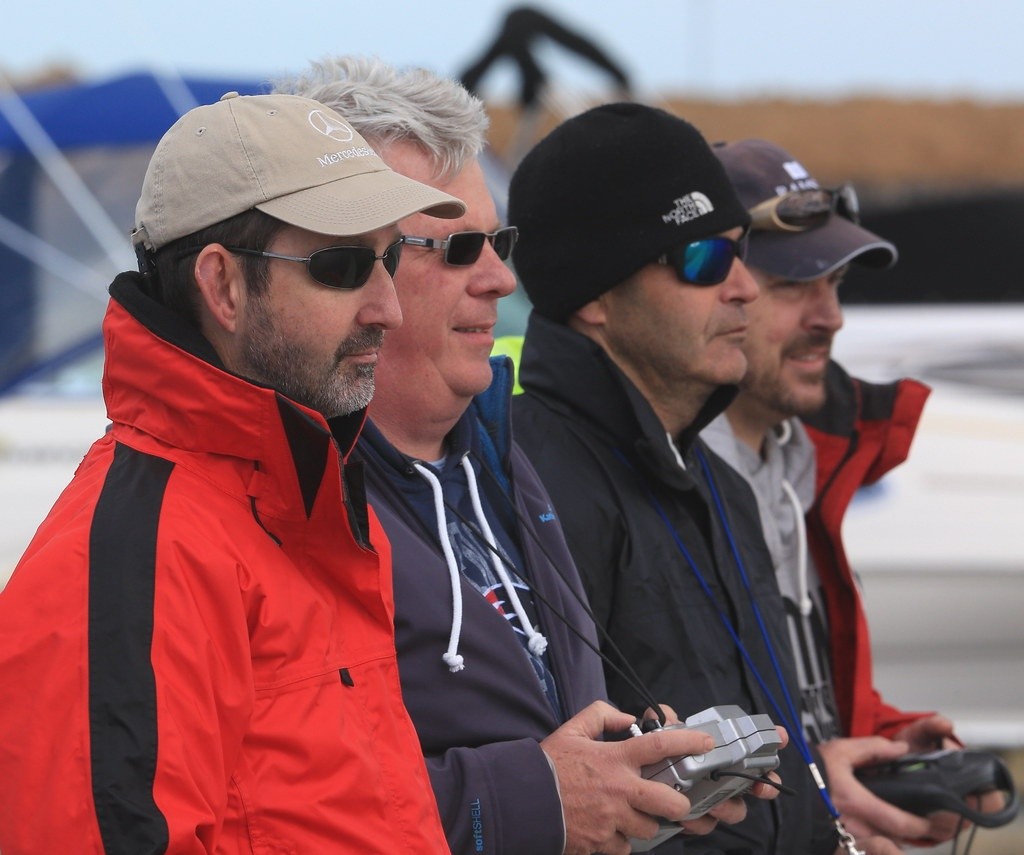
[[614, 448, 862, 855]]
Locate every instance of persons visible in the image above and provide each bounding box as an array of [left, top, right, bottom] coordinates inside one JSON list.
[[0, 94, 466, 855], [702, 138, 1004, 855], [272, 56, 788, 855], [507, 102, 838, 855]]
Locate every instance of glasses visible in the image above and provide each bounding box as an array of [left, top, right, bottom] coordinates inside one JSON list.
[[404, 226, 519, 267], [654, 233, 745, 287], [175, 235, 405, 290], [746, 184, 861, 233]]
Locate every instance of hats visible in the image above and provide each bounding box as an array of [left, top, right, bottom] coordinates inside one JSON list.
[[508, 101, 750, 321], [709, 138, 898, 281], [131, 92, 468, 273]]
